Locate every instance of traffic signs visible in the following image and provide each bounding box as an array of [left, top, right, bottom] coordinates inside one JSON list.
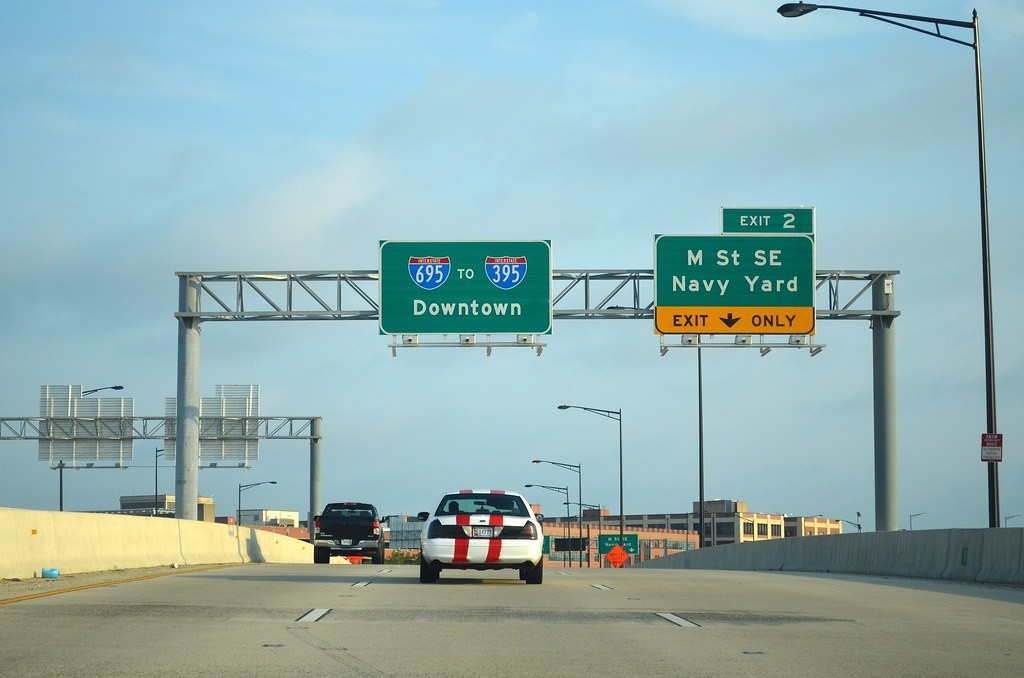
[[653, 233, 816, 338]]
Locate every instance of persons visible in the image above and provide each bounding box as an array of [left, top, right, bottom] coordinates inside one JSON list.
[[449, 501, 459, 512]]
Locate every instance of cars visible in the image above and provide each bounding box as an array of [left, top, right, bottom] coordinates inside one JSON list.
[[418, 490, 543, 584]]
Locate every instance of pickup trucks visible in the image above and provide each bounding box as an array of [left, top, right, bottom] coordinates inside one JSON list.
[[312, 502, 390, 565]]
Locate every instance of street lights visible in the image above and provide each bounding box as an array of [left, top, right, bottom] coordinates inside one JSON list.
[[558, 405, 624, 569], [239, 481, 277, 526], [532, 460, 582, 567], [60, 386, 126, 511], [779, 2, 1000, 530], [525, 484, 572, 568], [563, 502, 602, 568]]
